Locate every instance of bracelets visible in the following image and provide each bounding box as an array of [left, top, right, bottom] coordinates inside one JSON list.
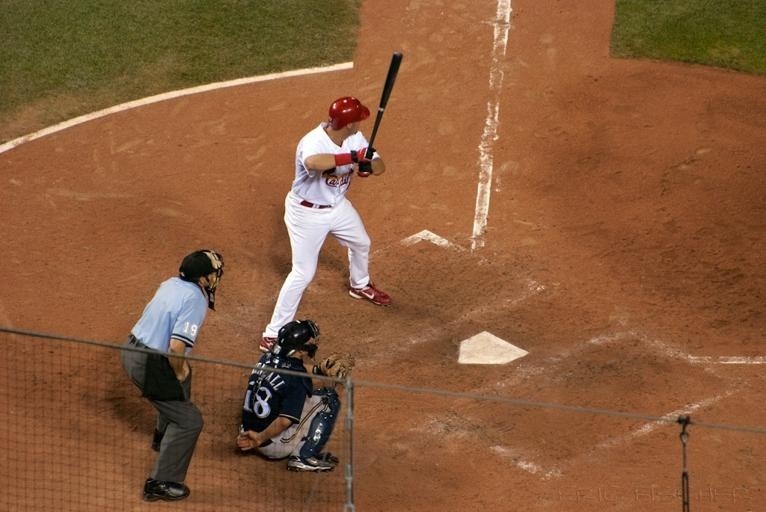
[[335, 153, 354, 166]]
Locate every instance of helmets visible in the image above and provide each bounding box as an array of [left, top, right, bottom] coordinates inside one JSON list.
[[180, 249, 225, 295], [274, 320, 320, 358], [327, 97, 371, 130]]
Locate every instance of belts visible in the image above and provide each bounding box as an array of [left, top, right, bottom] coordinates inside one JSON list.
[[301, 200, 331, 209]]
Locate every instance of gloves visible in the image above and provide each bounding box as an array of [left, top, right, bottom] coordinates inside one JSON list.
[[352, 147, 372, 177]]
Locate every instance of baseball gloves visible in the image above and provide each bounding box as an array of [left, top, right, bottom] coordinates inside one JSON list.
[[321, 352, 355, 384]]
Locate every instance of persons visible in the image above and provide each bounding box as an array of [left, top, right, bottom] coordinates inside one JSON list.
[[259, 97, 391, 352], [120, 249, 224, 502], [236, 320, 356, 472]]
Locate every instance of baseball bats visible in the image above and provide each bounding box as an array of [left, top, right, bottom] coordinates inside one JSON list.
[[355, 52, 403, 177]]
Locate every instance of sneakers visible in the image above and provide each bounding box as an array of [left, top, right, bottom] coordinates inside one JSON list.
[[142, 477, 189, 502], [152, 428, 166, 451], [348, 281, 392, 306], [259, 337, 277, 353], [287, 453, 335, 474]]
